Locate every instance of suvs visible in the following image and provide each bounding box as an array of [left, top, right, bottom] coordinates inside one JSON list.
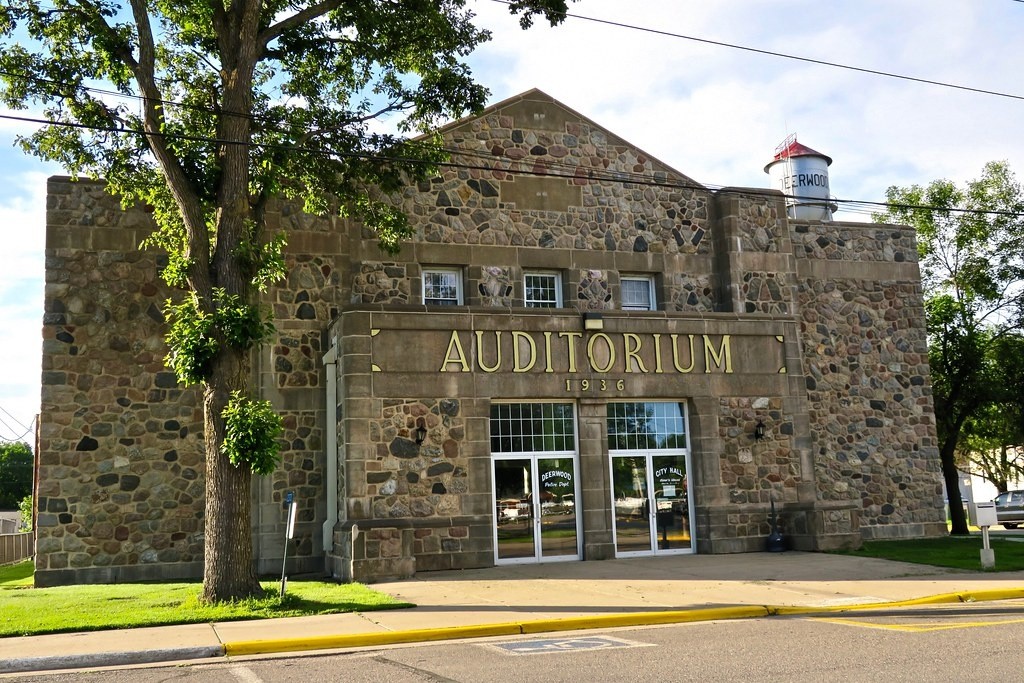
[[976, 489, 1024, 530]]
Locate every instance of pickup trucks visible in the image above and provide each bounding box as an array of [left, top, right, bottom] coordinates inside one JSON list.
[[646, 487, 687, 516]]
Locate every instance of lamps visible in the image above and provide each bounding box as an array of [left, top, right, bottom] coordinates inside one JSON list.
[[582, 312, 604, 330]]
